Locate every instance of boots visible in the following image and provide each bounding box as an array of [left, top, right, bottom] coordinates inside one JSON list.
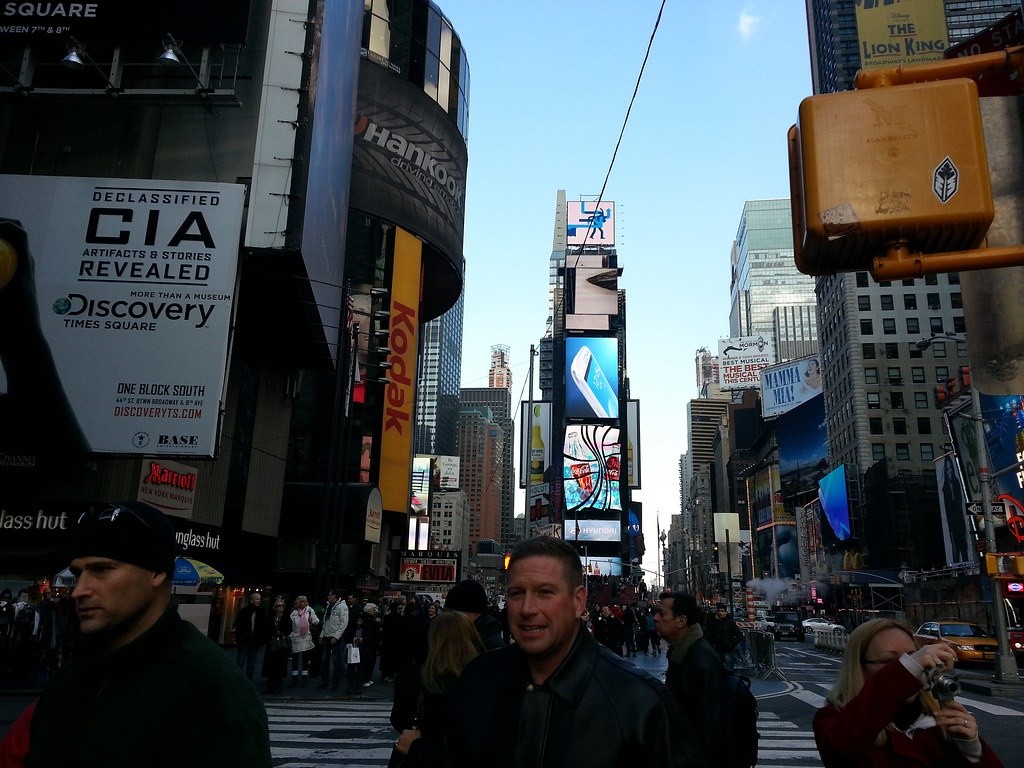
[[288, 675, 298, 688], [302, 675, 309, 688]]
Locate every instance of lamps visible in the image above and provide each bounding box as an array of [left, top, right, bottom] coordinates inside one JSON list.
[[293, 278, 393, 385], [157, 33, 215, 113], [61, 36, 123, 97], [949, 563, 952, 568]]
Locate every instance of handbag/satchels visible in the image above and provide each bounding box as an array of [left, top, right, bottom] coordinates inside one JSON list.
[[310, 622, 322, 639], [348, 644, 360, 663]]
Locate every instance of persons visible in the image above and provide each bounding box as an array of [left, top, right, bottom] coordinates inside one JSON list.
[[444, 578, 503, 651], [0, 583, 742, 699], [440, 539, 702, 768], [0, 499, 272, 768], [390, 611, 486, 768], [812, 617, 1005, 768], [801, 359, 824, 404], [653, 592, 745, 768], [942, 456, 968, 563]]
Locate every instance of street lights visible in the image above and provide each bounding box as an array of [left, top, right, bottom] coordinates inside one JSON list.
[[713, 547, 734, 620], [916, 336, 1019, 683], [581, 546, 588, 590]]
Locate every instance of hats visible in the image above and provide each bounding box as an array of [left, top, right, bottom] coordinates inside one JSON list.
[[67, 499, 179, 580], [444, 579, 487, 613]]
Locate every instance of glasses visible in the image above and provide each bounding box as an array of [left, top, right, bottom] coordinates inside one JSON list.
[[277, 605, 284, 607], [862, 658, 900, 666]]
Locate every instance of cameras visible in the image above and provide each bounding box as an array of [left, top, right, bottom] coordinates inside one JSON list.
[[931, 669, 962, 701]]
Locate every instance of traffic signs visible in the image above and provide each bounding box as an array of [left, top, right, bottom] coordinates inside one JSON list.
[[963, 502, 1005, 516]]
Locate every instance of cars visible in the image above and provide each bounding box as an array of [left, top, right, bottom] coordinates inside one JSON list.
[[802, 618, 845, 635], [755, 615, 775, 631], [912, 619, 1000, 662]]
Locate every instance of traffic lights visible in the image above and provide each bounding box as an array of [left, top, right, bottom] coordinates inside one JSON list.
[[980, 555, 997, 576], [589, 565, 592, 573]]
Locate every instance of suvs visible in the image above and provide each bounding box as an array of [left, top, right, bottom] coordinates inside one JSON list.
[[773, 610, 806, 641]]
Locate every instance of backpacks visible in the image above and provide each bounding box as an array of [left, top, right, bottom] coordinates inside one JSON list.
[[700, 666, 761, 768]]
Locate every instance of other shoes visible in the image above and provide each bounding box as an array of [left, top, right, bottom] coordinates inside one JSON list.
[[633, 654, 636, 657], [624, 655, 631, 657], [363, 681, 374, 687], [384, 677, 394, 684]]
